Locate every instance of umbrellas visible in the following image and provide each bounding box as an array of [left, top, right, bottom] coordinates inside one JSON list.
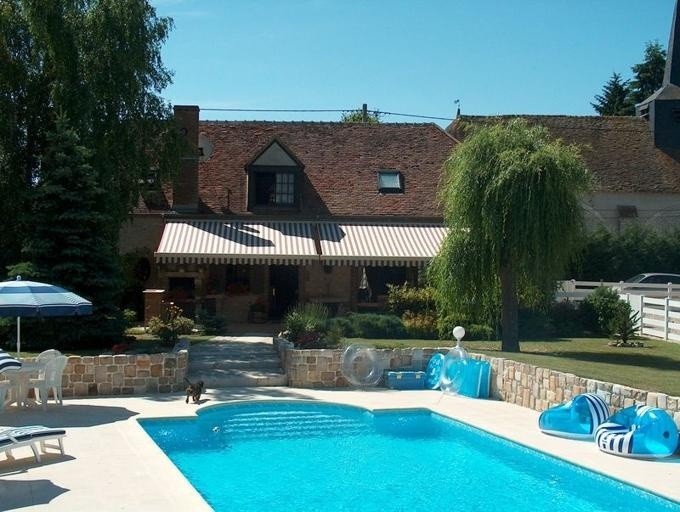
[[0, 275, 94, 361]]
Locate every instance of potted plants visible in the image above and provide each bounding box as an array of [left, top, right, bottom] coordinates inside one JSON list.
[[248, 304, 268, 323]]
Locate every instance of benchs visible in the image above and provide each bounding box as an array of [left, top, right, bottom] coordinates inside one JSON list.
[[384, 371, 425, 390]]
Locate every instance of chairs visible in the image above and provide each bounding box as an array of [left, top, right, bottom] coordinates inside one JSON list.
[[0, 425, 65, 463], [539, 393, 611, 440], [594, 402, 680, 459], [0, 349, 68, 412]]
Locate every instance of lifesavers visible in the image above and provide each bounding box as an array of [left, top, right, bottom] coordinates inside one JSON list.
[[440, 346, 470, 396], [341, 342, 384, 389], [425, 352, 446, 390]]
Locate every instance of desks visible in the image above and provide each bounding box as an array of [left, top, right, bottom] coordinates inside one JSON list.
[[307, 297, 351, 317]]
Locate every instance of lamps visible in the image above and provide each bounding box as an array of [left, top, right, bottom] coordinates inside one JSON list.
[[453, 326, 465, 348]]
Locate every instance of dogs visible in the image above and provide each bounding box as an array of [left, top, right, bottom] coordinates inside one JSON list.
[[183, 377, 204, 404]]
